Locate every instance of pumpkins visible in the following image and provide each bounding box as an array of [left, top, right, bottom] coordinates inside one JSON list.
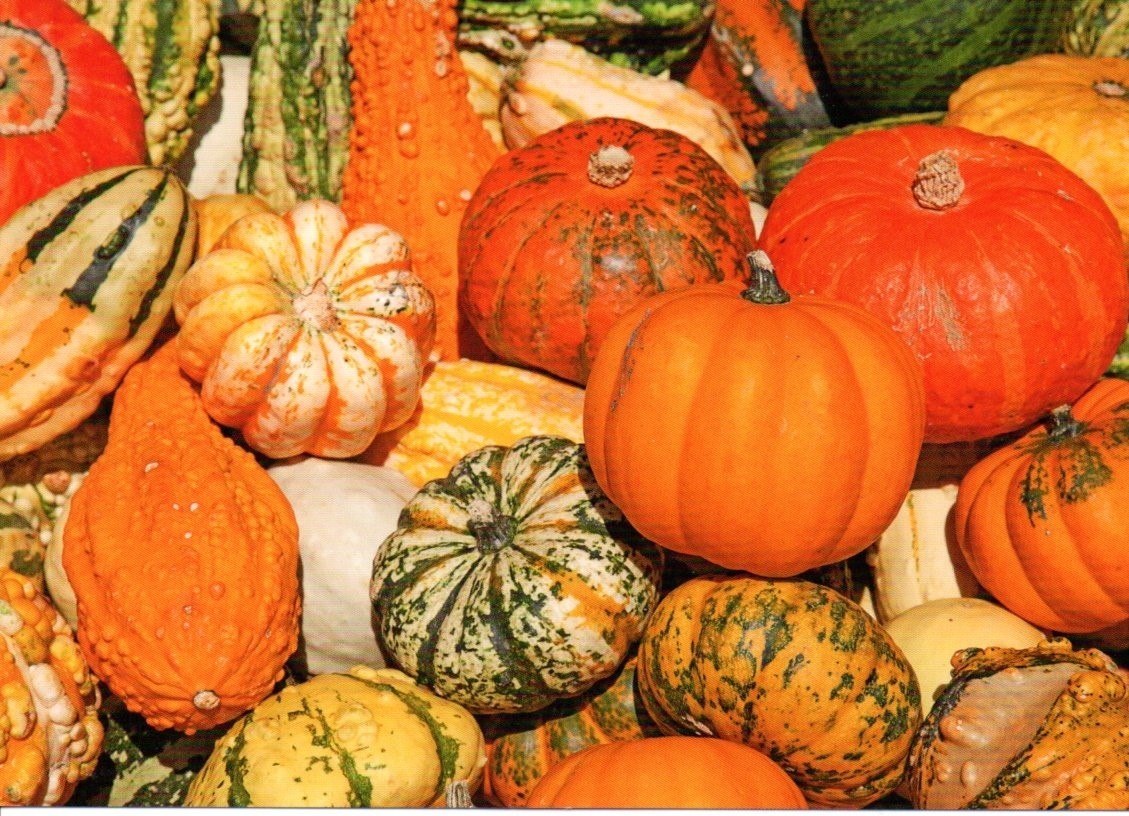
[[0, 0, 1128, 810]]
